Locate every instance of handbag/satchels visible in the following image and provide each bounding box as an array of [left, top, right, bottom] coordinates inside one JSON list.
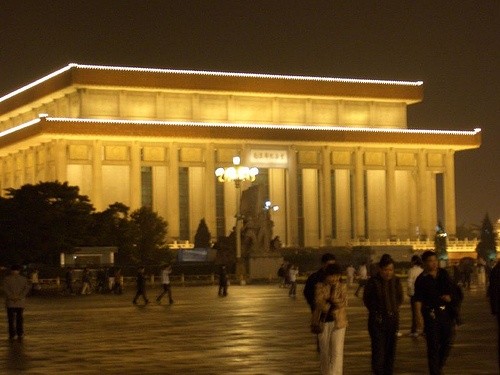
[[312, 307, 325, 334]]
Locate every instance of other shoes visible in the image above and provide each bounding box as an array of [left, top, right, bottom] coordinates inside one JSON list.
[[406, 332, 419, 337]]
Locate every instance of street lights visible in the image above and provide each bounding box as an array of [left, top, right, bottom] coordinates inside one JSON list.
[[264, 201, 280, 251], [214, 156, 260, 282]]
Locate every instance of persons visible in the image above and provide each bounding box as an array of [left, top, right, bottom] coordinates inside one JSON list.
[[0, 253, 500, 356], [315, 265, 348, 375], [415, 251, 463, 373], [361, 253, 404, 375]]
